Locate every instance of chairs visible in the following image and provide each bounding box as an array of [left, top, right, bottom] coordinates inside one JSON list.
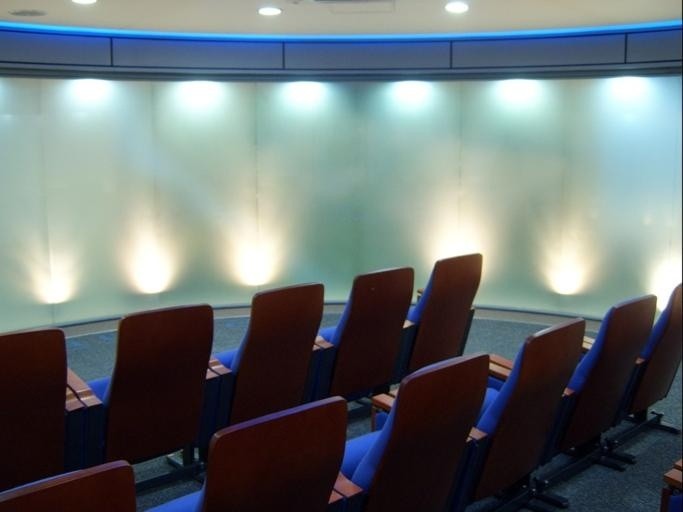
[[408, 254, 482, 371], [67, 304, 214, 468], [583, 282, 682, 464], [661, 454, 682, 509], [488, 295, 654, 475], [2, 327, 86, 493], [3, 459, 136, 508], [313, 267, 416, 403], [387, 313, 586, 509], [207, 282, 326, 426], [333, 351, 490, 508], [150, 396, 346, 509]]
